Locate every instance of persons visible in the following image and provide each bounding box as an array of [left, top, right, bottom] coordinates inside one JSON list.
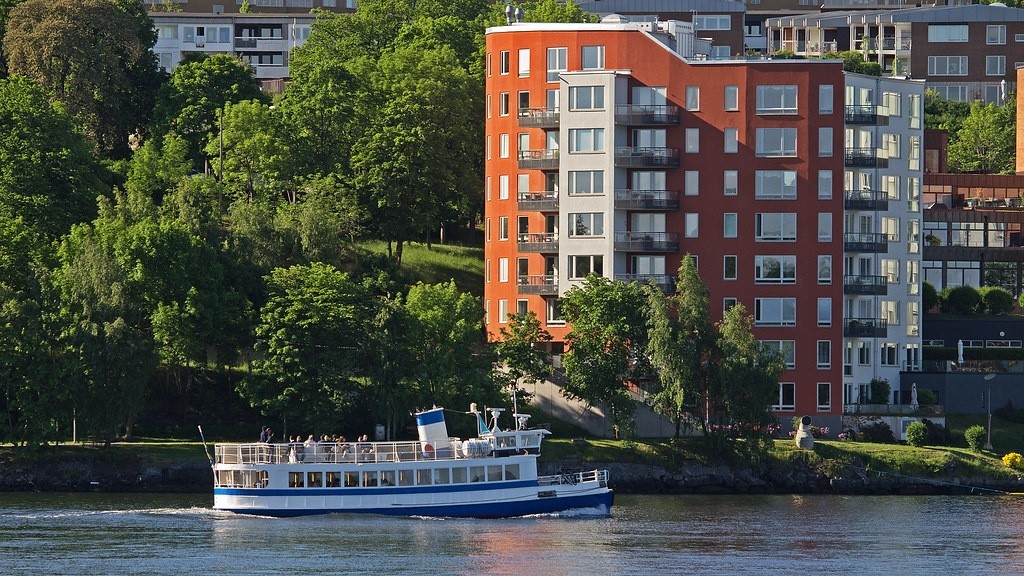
[[291, 472, 395, 488], [286, 434, 372, 463], [260, 425, 275, 464]]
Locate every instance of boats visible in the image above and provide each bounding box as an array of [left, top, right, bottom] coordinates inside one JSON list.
[[198, 383, 615, 520]]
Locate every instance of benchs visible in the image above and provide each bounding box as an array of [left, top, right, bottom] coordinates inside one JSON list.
[[289, 446, 388, 463]]
